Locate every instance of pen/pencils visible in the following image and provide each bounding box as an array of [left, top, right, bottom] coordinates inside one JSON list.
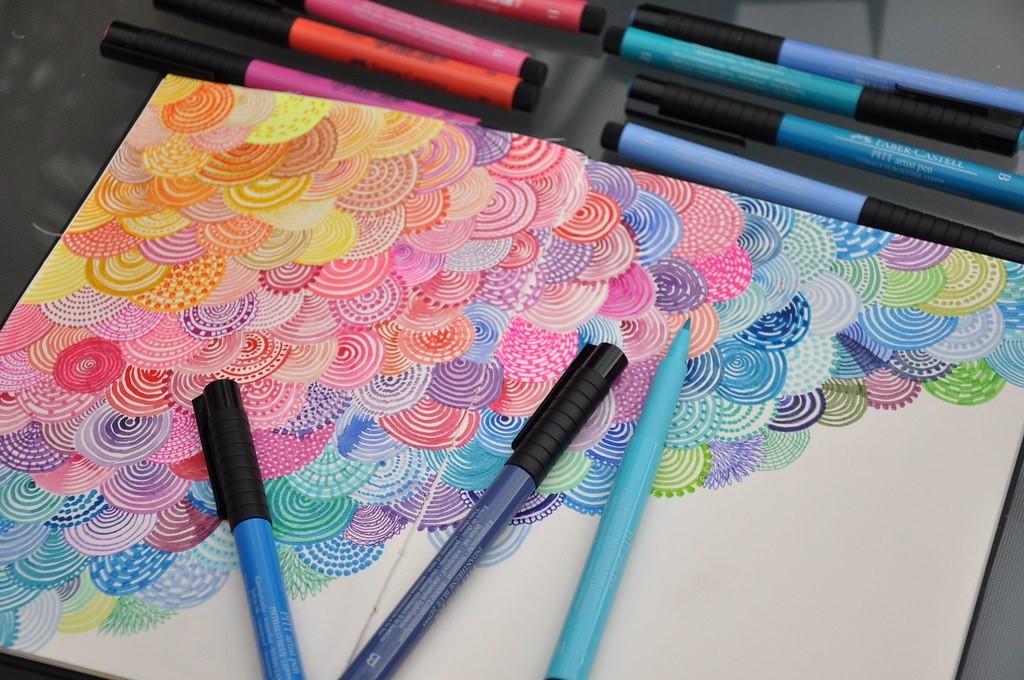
[[339, 341, 630, 680], [543, 315, 694, 680], [98, 0, 606, 130], [601, 0, 1024, 267], [190, 379, 311, 679]]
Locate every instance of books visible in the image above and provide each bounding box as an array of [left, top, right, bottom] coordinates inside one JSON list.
[[0, 75, 1024, 680]]
